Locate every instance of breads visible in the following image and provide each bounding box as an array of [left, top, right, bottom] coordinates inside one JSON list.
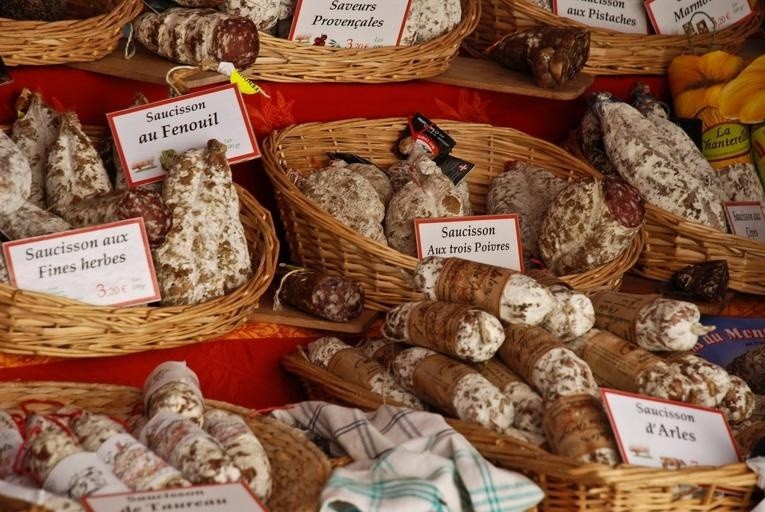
[[305, 129, 644, 255], [130, 9, 260, 70], [306, 256, 763, 471], [0, 361, 277, 511], [584, 86, 763, 235], [0, 87, 251, 305]]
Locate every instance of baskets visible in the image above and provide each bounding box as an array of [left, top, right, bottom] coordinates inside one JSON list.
[[0, 125, 279, 358], [244, 1, 480, 84], [561, 137, 765, 300], [0, 382, 332, 511], [462, 0, 762, 74], [1, 2, 764, 512], [259, 118, 646, 315], [282, 351, 759, 511], [0, 0, 144, 64]]
